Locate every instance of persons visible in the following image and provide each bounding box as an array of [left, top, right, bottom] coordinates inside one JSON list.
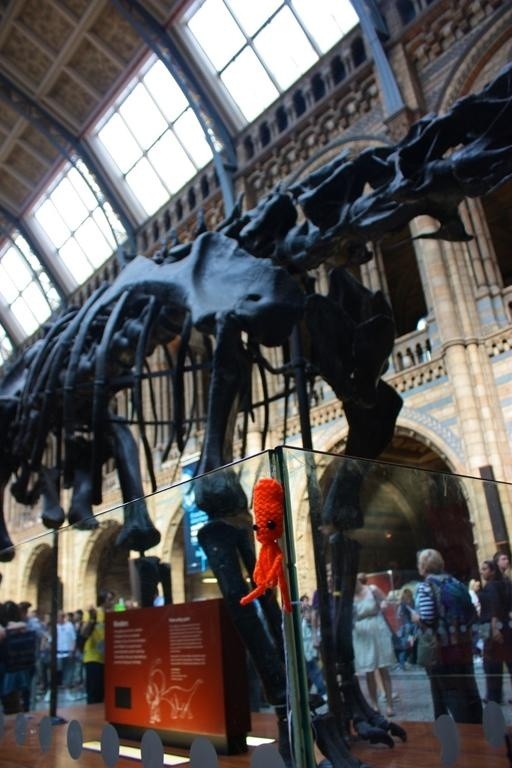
[[294, 549, 512, 724], [0, 589, 139, 715]]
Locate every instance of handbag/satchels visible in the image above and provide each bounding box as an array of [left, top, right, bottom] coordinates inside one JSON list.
[[392, 633, 404, 652]]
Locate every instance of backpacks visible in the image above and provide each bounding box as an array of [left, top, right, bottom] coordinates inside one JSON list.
[[425, 576, 477, 634]]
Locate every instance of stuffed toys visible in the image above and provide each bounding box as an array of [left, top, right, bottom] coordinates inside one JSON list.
[[240, 478, 291, 615]]
